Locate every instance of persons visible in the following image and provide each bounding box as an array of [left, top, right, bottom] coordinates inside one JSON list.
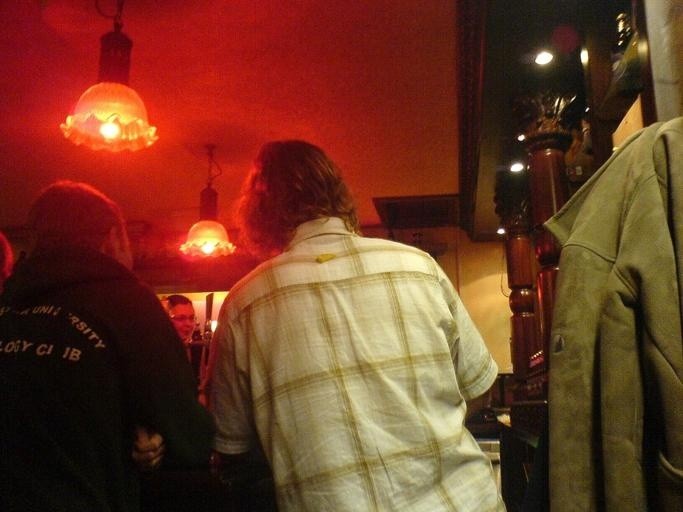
[[161, 296, 212, 417], [199, 140, 509, 511], [0, 183, 216, 512]]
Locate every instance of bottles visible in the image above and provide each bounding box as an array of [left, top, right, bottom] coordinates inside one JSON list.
[[203, 319, 212, 341], [192, 318, 203, 341]]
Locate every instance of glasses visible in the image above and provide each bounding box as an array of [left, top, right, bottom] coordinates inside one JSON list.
[[171, 315, 197, 323]]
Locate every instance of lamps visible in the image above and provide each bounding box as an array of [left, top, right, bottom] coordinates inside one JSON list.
[[180, 144, 237, 256], [59, 0, 159, 151]]
